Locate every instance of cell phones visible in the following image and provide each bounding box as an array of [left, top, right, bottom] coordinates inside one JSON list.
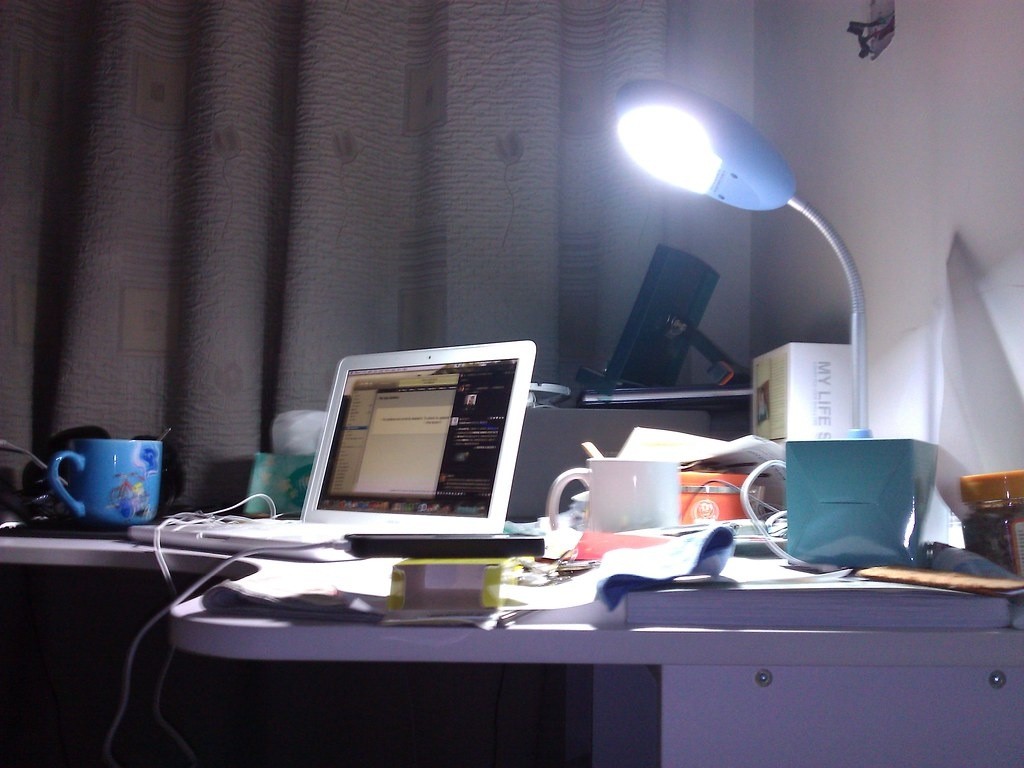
[[343, 534, 545, 558]]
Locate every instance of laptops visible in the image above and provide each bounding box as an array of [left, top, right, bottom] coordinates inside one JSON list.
[[128, 341, 536, 561]]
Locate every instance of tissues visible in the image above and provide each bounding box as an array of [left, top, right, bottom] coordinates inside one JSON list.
[[242, 410, 325, 514]]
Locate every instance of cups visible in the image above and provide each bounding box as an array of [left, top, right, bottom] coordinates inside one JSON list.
[[545, 457, 680, 532], [47, 439, 163, 526]]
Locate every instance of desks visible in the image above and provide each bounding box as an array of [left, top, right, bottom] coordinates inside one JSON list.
[[0, 525, 1024, 768]]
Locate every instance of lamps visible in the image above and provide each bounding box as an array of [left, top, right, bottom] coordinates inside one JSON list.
[[601, 79, 865, 554]]
